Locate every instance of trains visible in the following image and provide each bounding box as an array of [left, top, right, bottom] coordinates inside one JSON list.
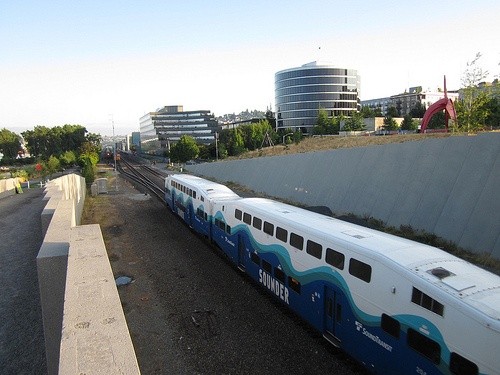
[[164, 171, 500, 374]]
